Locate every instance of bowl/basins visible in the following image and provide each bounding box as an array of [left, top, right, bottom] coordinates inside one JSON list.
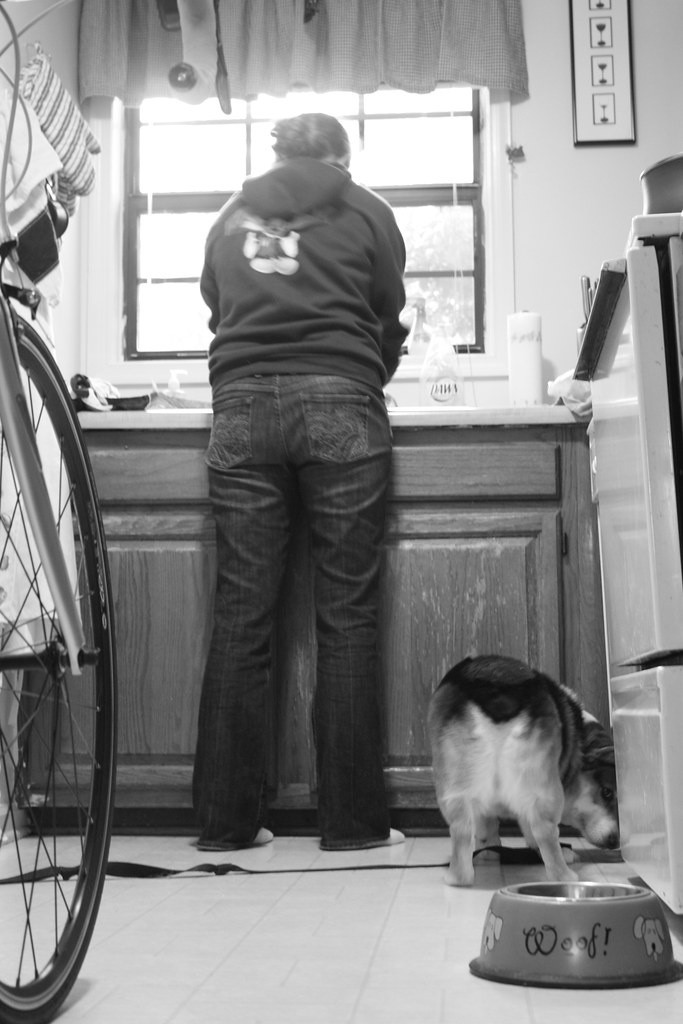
[[469, 882, 682, 988]]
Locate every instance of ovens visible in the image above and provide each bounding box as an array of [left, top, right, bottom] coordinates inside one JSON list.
[[573, 233, 683, 914]]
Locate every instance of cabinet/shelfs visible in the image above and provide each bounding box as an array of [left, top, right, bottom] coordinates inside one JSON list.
[[17, 429, 606, 809]]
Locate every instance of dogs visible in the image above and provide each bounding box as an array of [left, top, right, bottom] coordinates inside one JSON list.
[[428, 655, 621, 887]]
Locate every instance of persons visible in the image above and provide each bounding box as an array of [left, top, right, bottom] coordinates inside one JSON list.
[[192, 113, 410, 850]]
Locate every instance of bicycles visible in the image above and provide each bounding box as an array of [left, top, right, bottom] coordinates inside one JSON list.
[[0, 0, 121, 1024]]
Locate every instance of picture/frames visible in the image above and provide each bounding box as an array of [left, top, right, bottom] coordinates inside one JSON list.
[[568, 0, 637, 146]]
